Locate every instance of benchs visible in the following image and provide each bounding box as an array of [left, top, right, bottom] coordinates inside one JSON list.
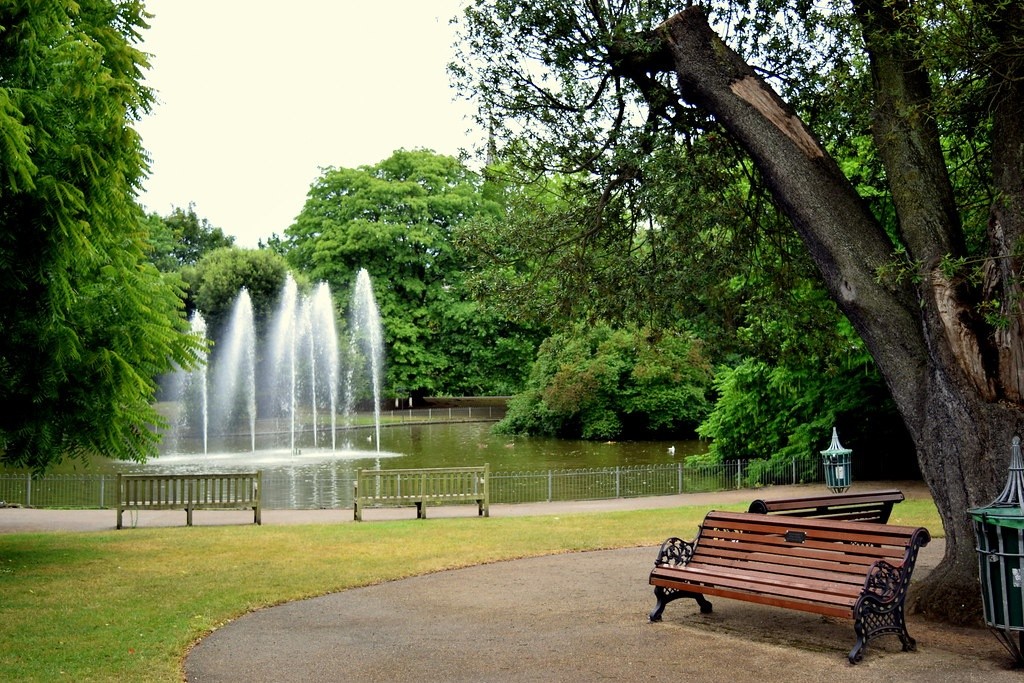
[[353, 463, 490, 522], [116, 468, 263, 529], [646, 508, 932, 664], [745, 487, 906, 548]]
